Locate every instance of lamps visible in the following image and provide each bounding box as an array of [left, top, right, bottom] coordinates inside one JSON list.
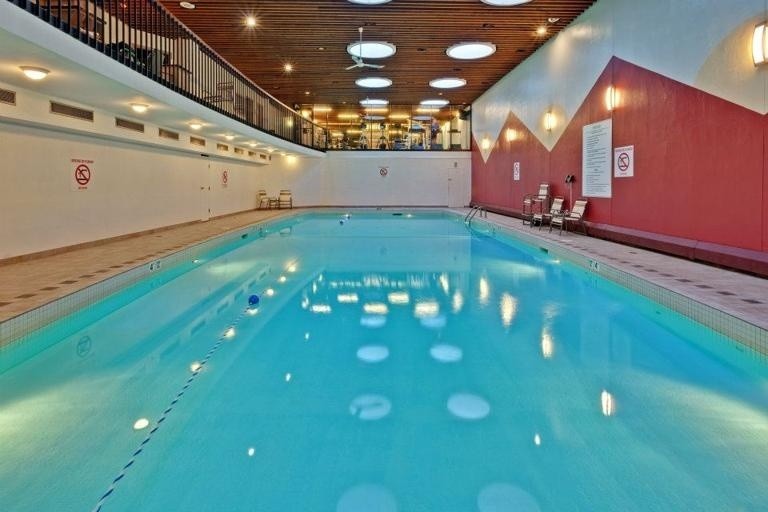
[[748, 18, 766, 68], [20, 66, 49, 81], [353, 76, 392, 88], [444, 42, 496, 61], [345, 40, 396, 59], [542, 111, 555, 131], [428, 77, 466, 89], [604, 84, 617, 112]]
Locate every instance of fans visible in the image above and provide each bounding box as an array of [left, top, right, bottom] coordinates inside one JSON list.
[[344, 26, 385, 72]]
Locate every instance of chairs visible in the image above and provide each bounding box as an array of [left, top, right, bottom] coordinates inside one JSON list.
[[522, 182, 591, 237], [201, 80, 236, 114], [255, 188, 293, 211]]
